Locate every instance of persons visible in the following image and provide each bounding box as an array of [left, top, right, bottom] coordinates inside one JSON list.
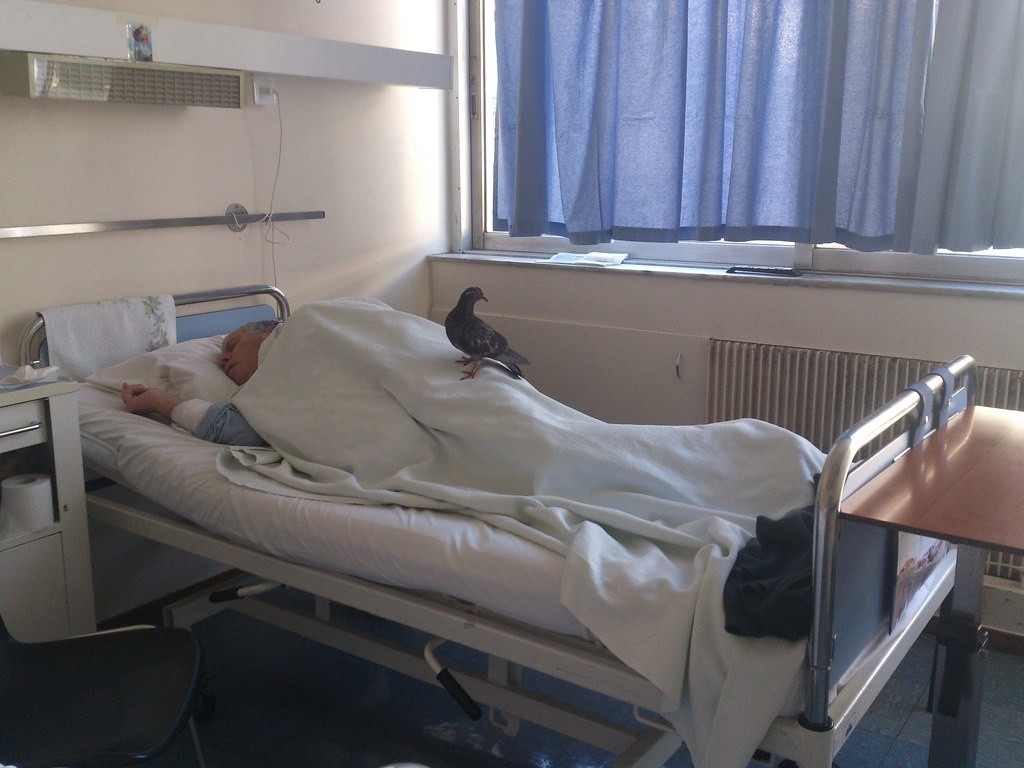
[[121, 320, 277, 446]]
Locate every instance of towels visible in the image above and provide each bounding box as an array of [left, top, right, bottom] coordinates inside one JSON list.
[[35, 293, 178, 383]]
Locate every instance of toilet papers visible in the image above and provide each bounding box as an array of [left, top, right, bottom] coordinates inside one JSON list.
[[0, 473, 55, 534]]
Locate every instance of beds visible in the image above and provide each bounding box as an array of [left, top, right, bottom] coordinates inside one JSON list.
[[21, 284, 978, 768]]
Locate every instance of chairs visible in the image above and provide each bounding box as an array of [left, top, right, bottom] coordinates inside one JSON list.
[[0, 615, 207, 768]]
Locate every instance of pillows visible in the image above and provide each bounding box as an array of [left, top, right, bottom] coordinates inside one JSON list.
[[83, 334, 239, 403]]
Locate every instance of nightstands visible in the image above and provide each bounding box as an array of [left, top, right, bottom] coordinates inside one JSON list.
[[0, 366, 98, 640]]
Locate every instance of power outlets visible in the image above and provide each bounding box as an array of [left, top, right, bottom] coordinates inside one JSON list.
[[251, 76, 277, 106]]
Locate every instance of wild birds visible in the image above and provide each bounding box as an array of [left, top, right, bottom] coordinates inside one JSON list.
[[444, 286, 533, 382]]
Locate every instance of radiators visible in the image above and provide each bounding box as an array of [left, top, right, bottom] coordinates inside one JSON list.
[[705, 338, 1024, 582]]
